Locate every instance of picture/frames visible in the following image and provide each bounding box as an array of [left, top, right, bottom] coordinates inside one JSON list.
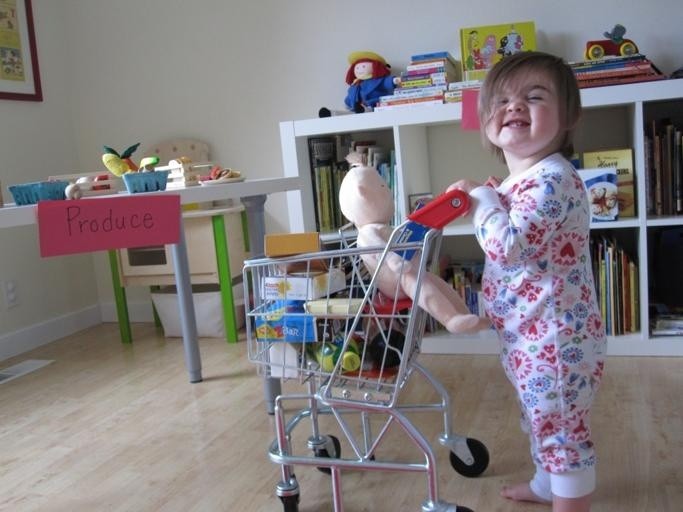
[[1, 0, 43, 102]]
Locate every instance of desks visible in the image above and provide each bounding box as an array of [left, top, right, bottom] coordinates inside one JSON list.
[[0, 177, 308, 384]]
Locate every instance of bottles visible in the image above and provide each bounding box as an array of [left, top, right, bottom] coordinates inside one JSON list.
[[305, 330, 361, 373]]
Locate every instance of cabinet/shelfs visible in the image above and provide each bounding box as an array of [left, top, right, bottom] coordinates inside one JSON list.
[[278, 79, 683, 358]]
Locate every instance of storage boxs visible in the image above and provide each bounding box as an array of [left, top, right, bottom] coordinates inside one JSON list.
[[114, 204, 248, 287]]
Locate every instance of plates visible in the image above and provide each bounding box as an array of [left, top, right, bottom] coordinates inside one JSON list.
[[196, 177, 246, 185]]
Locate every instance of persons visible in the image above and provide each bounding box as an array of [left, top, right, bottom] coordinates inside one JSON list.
[[443, 51, 608, 511]]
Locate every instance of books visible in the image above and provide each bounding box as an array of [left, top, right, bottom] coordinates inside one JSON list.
[[644, 118, 683, 220], [590, 233, 639, 336], [425, 255, 486, 333], [374, 52, 485, 112], [565, 54, 667, 88], [649, 301, 683, 336], [310, 136, 402, 233]]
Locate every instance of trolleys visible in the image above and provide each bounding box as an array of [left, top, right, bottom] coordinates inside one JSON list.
[[243, 190, 489, 512]]
